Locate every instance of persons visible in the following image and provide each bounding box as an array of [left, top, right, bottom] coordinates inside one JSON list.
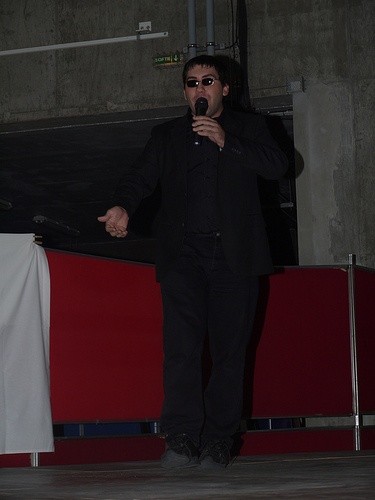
[[98, 55, 288, 468]]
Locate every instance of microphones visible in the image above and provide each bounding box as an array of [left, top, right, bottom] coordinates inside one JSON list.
[[193, 97, 208, 145]]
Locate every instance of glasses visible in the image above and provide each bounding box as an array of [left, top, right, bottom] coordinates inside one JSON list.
[[186, 77, 216, 88]]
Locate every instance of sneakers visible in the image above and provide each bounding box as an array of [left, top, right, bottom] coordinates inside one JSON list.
[[158, 434, 201, 468], [198, 433, 236, 469]]
